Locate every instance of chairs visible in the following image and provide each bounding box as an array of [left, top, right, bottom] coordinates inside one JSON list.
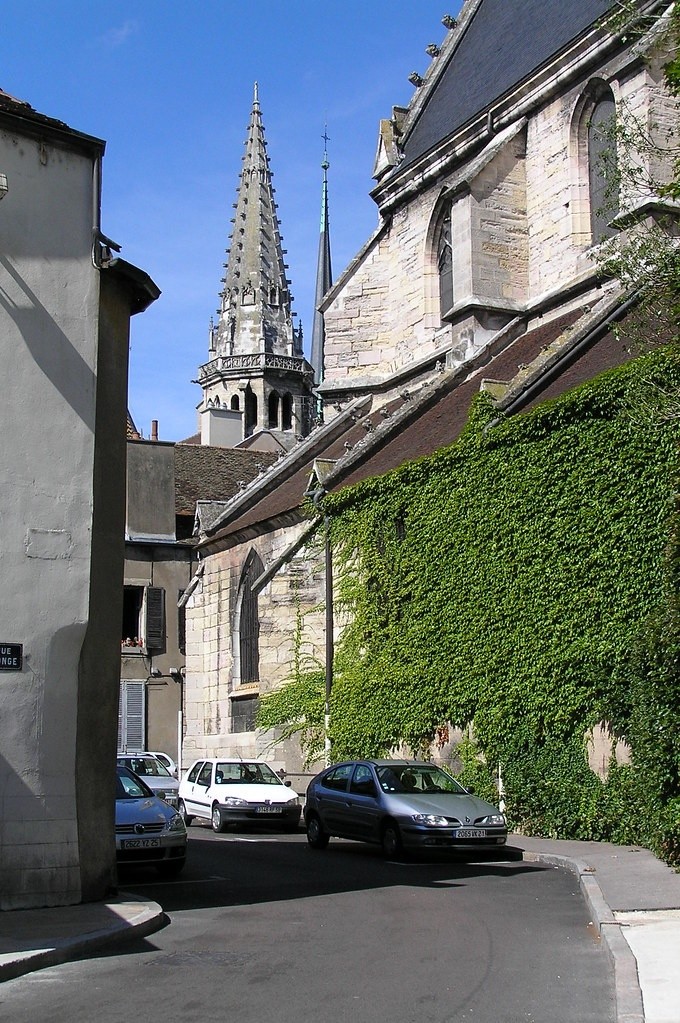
[[358, 774, 375, 794], [135, 766, 147, 774], [397, 774, 422, 790], [244, 770, 257, 781], [208, 769, 224, 783]]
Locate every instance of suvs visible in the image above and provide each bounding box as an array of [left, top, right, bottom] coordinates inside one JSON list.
[[116, 751, 180, 813]]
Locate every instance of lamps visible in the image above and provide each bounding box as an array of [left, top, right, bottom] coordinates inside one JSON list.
[[0, 174, 9, 202]]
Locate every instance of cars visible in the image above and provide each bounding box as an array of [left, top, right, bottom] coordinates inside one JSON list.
[[115, 765, 189, 869], [178, 757, 302, 834], [304, 759, 508, 859]]
